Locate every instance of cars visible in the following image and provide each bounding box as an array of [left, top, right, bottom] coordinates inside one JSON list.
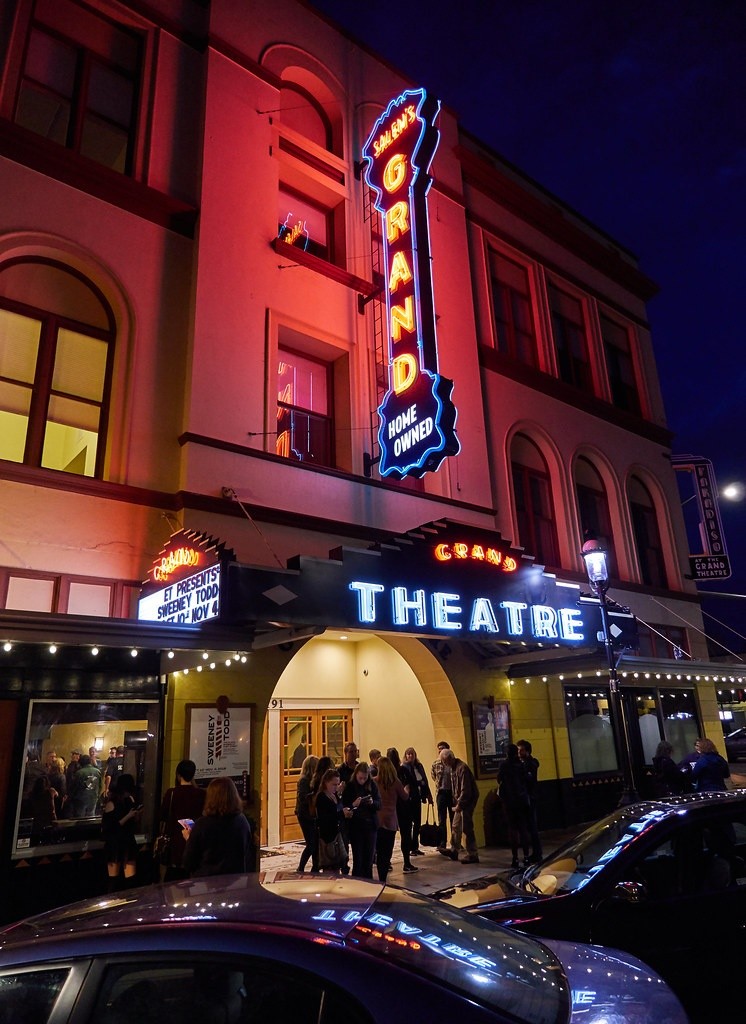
[[0, 789, 746, 1024], [723, 727, 746, 763]]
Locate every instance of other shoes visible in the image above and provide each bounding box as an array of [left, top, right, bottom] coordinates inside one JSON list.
[[403, 865, 418, 873], [416, 850, 424, 855], [439, 848, 458, 860], [461, 853, 479, 864], [529, 854, 542, 862], [411, 851, 417, 856], [511, 857, 519, 868], [524, 856, 530, 868]]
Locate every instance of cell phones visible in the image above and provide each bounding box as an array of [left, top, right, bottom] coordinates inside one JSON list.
[[361, 795, 371, 801], [185, 820, 194, 830], [136, 805, 144, 811], [350, 808, 357, 813]]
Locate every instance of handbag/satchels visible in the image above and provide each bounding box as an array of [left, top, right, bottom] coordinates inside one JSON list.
[[154, 834, 173, 857], [420, 824, 442, 846], [318, 832, 348, 869]]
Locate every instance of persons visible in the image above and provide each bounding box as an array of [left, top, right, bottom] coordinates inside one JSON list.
[[430, 742, 456, 850], [485, 711, 495, 750], [100, 775, 144, 891], [27, 776, 59, 845], [439, 749, 480, 864], [652, 740, 689, 797], [20, 743, 125, 818], [182, 777, 252, 883], [294, 742, 432, 882], [142, 761, 207, 883], [677, 738, 730, 793], [496, 740, 543, 868], [292, 734, 306, 775]]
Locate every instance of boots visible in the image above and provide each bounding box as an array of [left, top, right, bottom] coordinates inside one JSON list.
[[125, 874, 137, 889], [106, 876, 120, 895]]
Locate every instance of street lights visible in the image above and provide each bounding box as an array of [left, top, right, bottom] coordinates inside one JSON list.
[[580, 538, 640, 804]]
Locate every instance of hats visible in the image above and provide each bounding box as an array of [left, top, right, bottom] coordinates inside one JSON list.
[[109, 774, 141, 793]]
